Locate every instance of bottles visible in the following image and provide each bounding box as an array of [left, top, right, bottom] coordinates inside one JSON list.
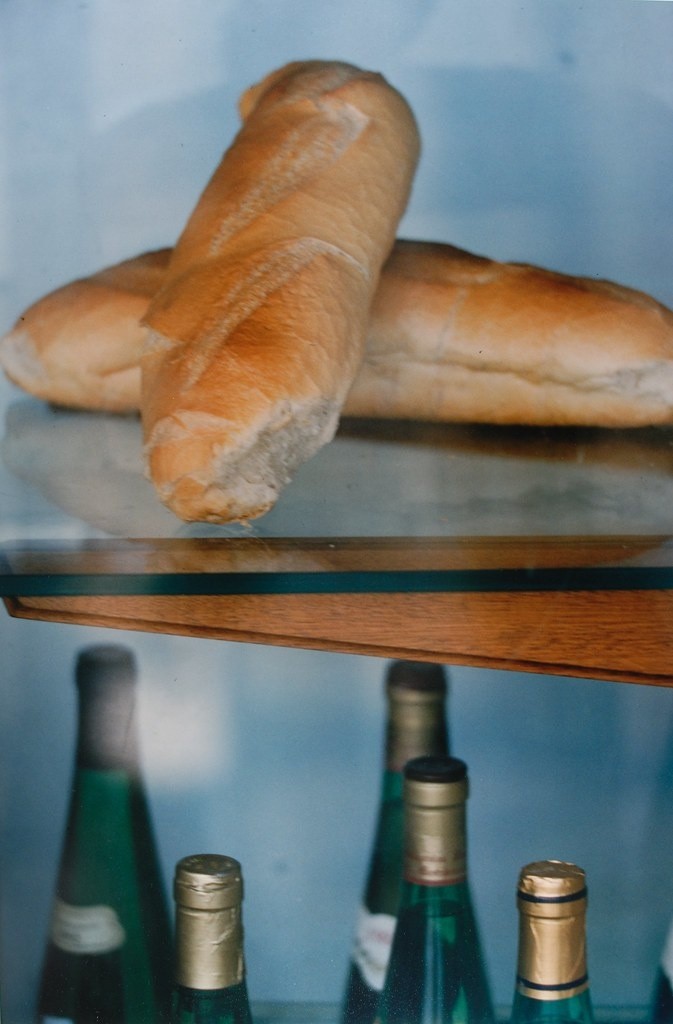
[[30, 642, 175, 1024], [343, 659, 450, 1024], [511, 860, 595, 1024], [375, 757, 496, 1024], [165, 854, 251, 1024]]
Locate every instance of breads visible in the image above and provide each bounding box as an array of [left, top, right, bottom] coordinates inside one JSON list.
[[143, 57, 422, 526], [0, 232, 673, 425]]
